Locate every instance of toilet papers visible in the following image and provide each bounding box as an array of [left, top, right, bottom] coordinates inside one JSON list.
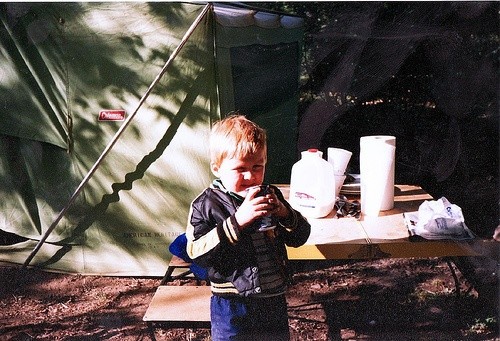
[[359, 135, 396, 213]]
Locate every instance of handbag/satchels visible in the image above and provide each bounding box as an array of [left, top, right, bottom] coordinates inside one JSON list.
[[416, 197, 465, 241]]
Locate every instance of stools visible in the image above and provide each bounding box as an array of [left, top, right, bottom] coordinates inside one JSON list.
[[143, 286, 211, 341], [160, 255, 210, 286]]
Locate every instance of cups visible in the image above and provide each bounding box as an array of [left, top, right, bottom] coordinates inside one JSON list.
[[328, 148, 352, 175], [334, 175, 346, 201]]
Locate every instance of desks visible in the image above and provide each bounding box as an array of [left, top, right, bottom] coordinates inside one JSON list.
[[271, 183, 500, 293]]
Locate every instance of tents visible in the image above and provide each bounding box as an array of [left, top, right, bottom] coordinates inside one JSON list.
[[0, 2, 305, 279]]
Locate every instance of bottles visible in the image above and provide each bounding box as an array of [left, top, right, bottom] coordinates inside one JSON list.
[[289, 149, 335, 219]]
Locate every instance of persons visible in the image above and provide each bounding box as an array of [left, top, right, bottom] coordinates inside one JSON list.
[[186, 112, 312, 341]]
